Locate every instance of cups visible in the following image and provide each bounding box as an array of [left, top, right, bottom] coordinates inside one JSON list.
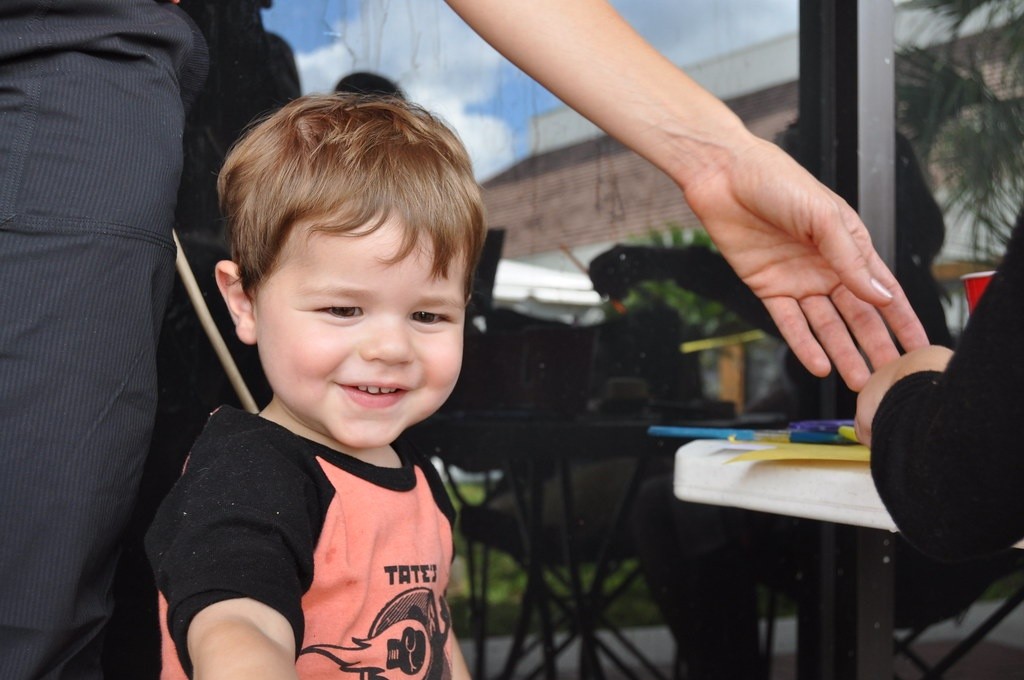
[[961, 269, 997, 316]]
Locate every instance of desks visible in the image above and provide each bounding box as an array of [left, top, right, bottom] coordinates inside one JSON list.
[[673, 433, 1024, 550], [427, 409, 789, 680]]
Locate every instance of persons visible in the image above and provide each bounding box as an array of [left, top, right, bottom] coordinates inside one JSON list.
[[853, 188, 1024, 680], [0, 1, 932, 680], [142, 91, 489, 680]]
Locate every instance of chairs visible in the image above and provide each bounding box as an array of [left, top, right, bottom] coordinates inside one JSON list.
[[427, 309, 777, 680]]
